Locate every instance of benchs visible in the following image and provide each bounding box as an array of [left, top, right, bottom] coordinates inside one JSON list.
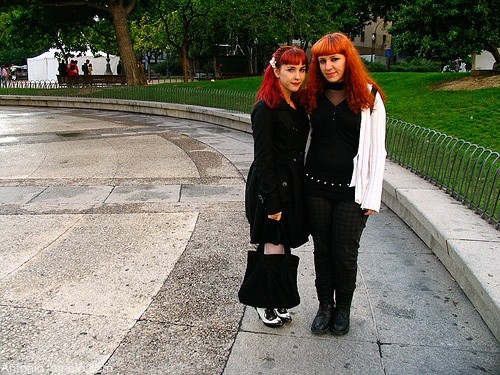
[[58, 75, 125, 86]]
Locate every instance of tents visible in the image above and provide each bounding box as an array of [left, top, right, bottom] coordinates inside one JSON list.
[[27, 45, 120, 83]]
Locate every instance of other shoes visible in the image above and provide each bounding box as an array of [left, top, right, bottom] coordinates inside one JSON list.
[[274, 308, 292, 323], [256, 308, 282, 327]]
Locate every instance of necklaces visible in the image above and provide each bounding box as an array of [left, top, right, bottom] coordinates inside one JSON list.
[[326, 82, 344, 90]]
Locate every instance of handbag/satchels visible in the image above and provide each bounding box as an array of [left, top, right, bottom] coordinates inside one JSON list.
[[239, 218, 300, 307]]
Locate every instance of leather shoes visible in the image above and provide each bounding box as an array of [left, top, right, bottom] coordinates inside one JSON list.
[[330, 308, 350, 335], [311, 304, 333, 334]]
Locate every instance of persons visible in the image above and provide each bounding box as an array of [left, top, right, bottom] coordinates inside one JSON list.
[[0, 59, 125, 85], [244, 46, 310, 327], [298, 32, 387, 336]]
